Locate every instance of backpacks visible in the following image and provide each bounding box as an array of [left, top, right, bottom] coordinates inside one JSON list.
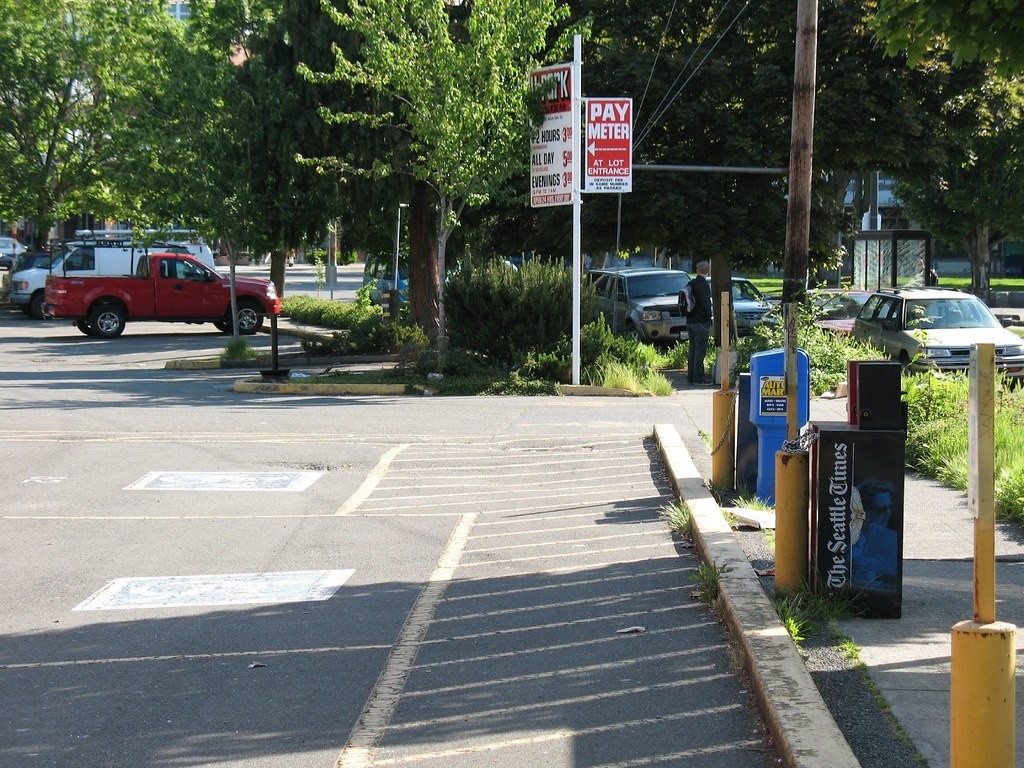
[[678, 278, 706, 317]]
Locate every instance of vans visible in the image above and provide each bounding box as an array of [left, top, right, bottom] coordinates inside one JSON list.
[[9, 228, 221, 320]]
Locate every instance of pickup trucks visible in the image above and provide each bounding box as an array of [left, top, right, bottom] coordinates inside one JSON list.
[[37, 252, 280, 335], [583, 266, 698, 343]]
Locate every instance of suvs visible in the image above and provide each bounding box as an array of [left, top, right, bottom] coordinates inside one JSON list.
[[848, 287, 1024, 382]]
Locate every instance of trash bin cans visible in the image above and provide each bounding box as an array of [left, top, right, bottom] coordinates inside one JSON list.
[[996, 291, 1009, 308], [990, 291, 996, 308]]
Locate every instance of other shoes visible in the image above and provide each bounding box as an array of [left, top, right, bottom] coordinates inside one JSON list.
[[693, 380, 713, 386], [687, 380, 693, 385]]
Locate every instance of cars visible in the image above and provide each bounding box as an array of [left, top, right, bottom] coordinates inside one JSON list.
[[761, 287, 870, 335], [694, 273, 781, 337], [362, 253, 452, 310], [0, 237, 28, 267]]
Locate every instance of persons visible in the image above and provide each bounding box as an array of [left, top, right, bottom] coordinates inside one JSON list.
[[687, 261, 714, 386], [911, 260, 926, 286]]
